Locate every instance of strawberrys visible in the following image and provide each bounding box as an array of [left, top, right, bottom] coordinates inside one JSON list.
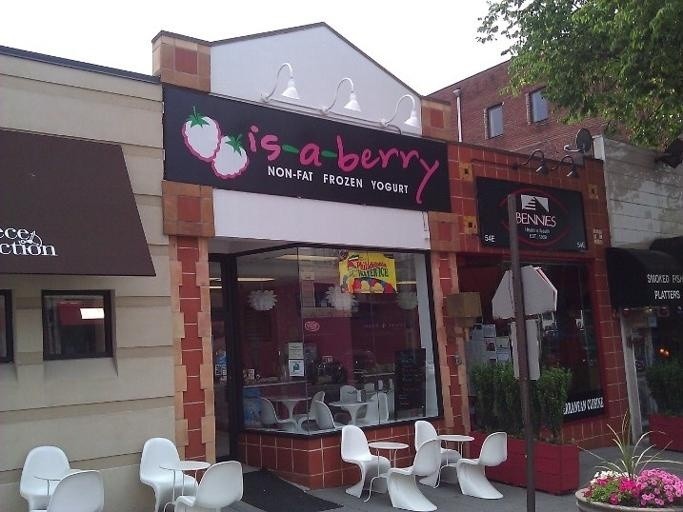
[[182, 106, 221, 163], [210, 134, 249, 180]]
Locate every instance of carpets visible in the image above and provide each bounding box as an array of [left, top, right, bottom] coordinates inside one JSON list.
[[242, 472, 343, 511]]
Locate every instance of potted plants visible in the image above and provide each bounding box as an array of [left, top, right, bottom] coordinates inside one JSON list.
[[467, 361, 579, 496], [645, 361, 682, 454]]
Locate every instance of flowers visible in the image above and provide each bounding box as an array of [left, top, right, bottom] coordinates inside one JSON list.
[[577, 410, 683, 509]]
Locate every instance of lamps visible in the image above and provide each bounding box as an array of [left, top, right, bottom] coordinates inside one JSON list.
[[323, 77, 361, 117], [522, 149, 549, 176], [261, 62, 300, 103], [552, 156, 579, 179], [381, 94, 420, 129]]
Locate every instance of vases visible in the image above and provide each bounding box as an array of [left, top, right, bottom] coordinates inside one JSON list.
[[575, 487, 683, 512]]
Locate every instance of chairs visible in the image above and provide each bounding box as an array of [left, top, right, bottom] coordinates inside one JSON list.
[[174, 461, 243, 512], [46, 470, 105, 512], [260, 386, 507, 512], [139, 438, 198, 512], [19, 446, 71, 512]]
